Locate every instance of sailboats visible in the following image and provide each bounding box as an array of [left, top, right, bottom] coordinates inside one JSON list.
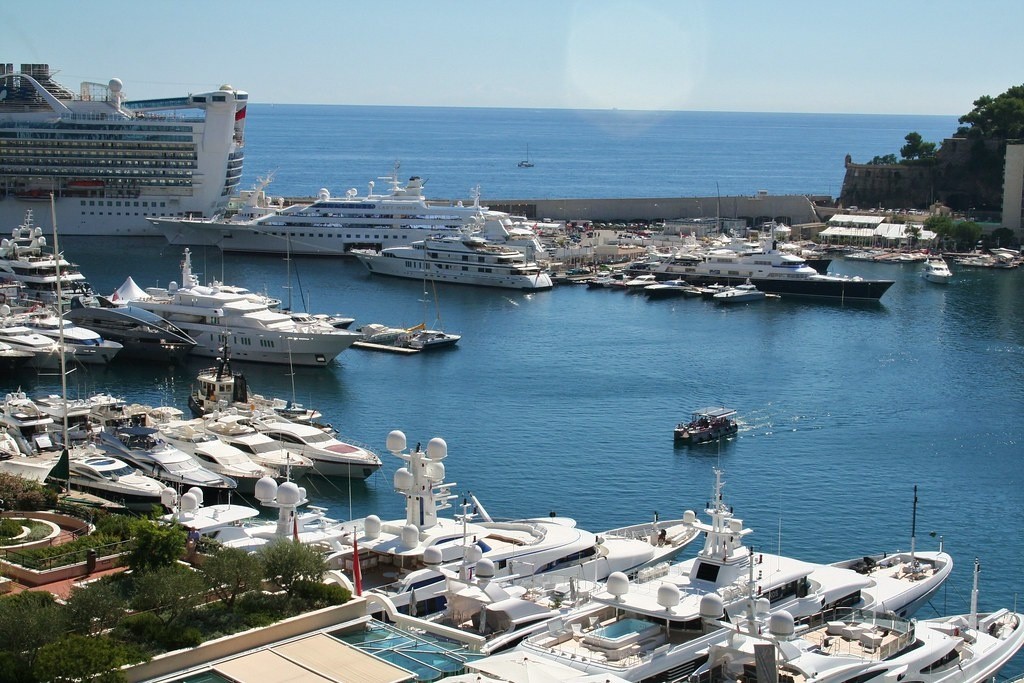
[[516, 142, 534, 168]]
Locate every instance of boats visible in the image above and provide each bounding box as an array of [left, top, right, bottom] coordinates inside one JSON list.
[[146, 168, 294, 246], [690, 558, 1024, 683], [0, 204, 1023, 660], [672, 402, 740, 448], [0, 63, 251, 237], [349, 227, 555, 291], [180, 160, 545, 257], [69, 250, 365, 370], [508, 486, 953, 683], [923, 247, 953, 286], [619, 215, 896, 302], [67, 180, 105, 191]]
